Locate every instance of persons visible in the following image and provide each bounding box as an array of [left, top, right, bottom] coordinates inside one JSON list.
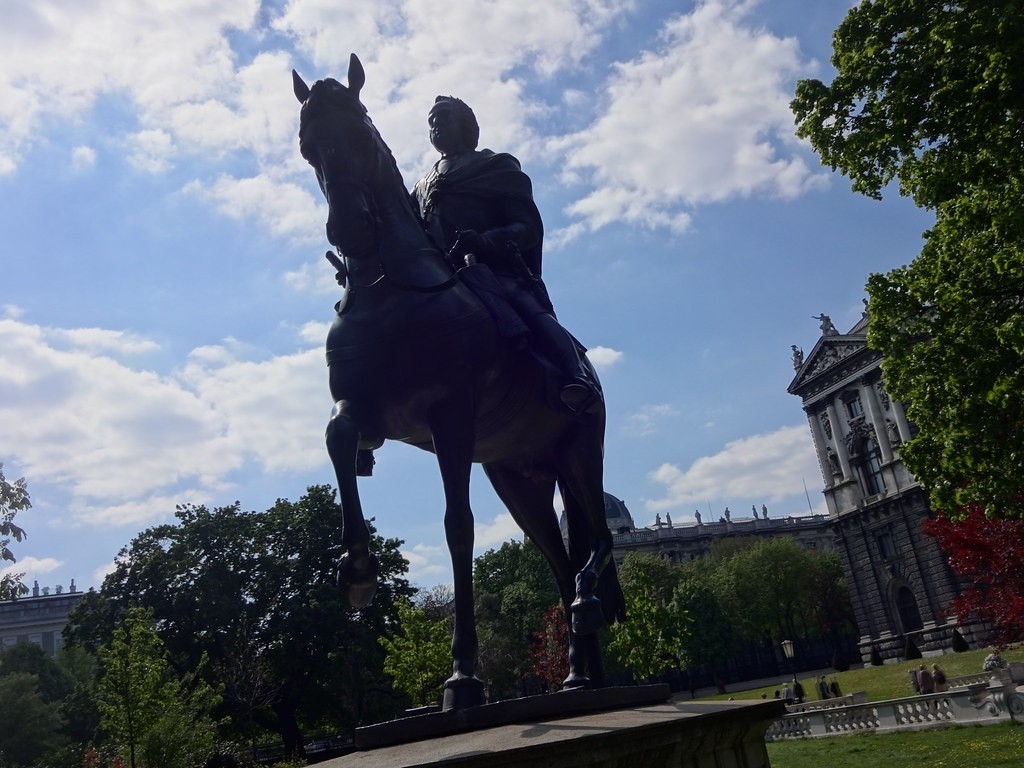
[[357, 96, 596, 478], [861, 298, 872, 321], [762, 693, 767, 698], [762, 505, 767, 519], [696, 511, 701, 525], [724, 508, 731, 522], [656, 513, 662, 528], [667, 513, 672, 527], [789, 345, 803, 369], [825, 446, 842, 474], [793, 680, 806, 712], [885, 418, 900, 446], [752, 506, 758, 519], [774, 690, 781, 698], [813, 313, 837, 333], [815, 676, 844, 699], [909, 663, 949, 710], [784, 683, 794, 705]]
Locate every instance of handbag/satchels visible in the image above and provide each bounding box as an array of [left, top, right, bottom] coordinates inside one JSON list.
[[934, 672, 945, 684]]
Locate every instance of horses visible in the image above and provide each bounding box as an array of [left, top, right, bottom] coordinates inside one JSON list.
[[289, 51, 621, 713]]
